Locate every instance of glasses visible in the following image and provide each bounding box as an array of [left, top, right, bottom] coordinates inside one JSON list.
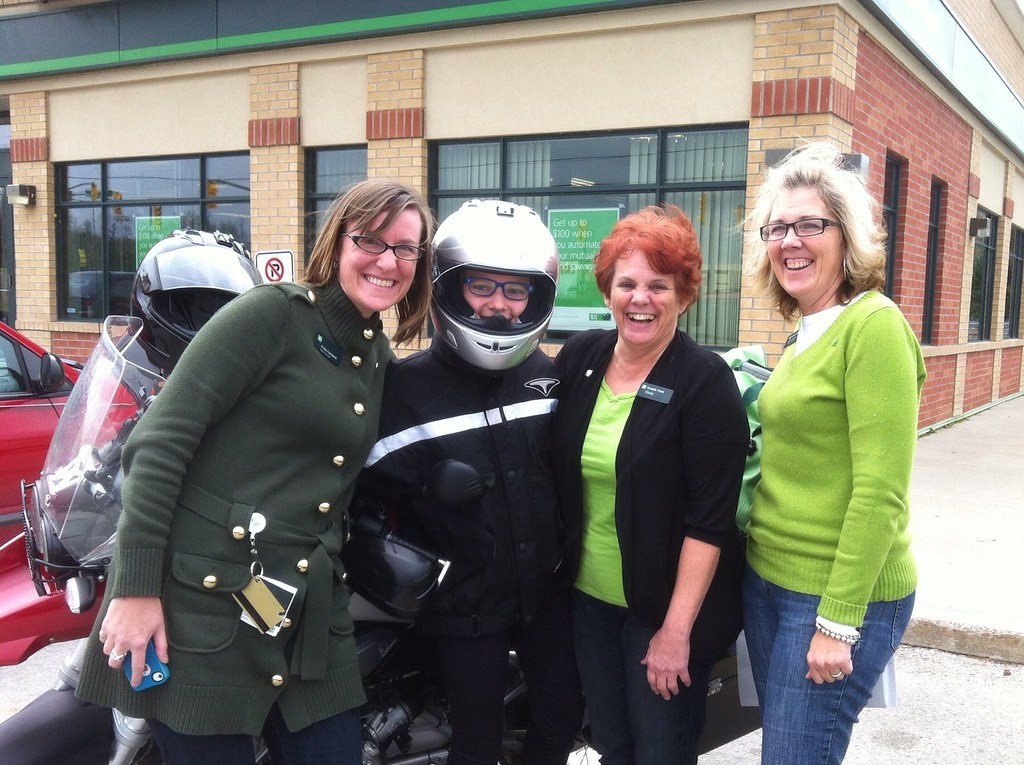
[[464, 275, 535, 302], [344, 232, 428, 261], [760, 218, 842, 240]]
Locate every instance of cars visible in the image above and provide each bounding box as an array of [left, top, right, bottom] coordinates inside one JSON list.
[[0, 319, 159, 575], [68, 270, 144, 318]]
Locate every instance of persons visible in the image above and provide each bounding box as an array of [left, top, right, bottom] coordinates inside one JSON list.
[[345, 199, 585, 765], [75, 179, 434, 765], [740, 143, 927, 765], [552, 201, 750, 765]]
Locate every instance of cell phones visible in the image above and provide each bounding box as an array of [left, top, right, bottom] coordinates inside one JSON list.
[[124, 640, 170, 692]]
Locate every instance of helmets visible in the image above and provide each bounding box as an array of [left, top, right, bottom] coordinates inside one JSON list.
[[130, 228, 266, 372], [430, 199, 560, 370]]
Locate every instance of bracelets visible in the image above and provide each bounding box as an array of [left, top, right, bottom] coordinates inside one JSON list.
[[815, 622, 857, 647]]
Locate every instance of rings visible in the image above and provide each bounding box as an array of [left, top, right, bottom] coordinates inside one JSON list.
[[100, 631, 107, 640], [110, 648, 127, 661], [830, 671, 843, 678]]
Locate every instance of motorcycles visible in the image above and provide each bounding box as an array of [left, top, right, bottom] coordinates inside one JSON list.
[[0, 317, 894, 765]]
